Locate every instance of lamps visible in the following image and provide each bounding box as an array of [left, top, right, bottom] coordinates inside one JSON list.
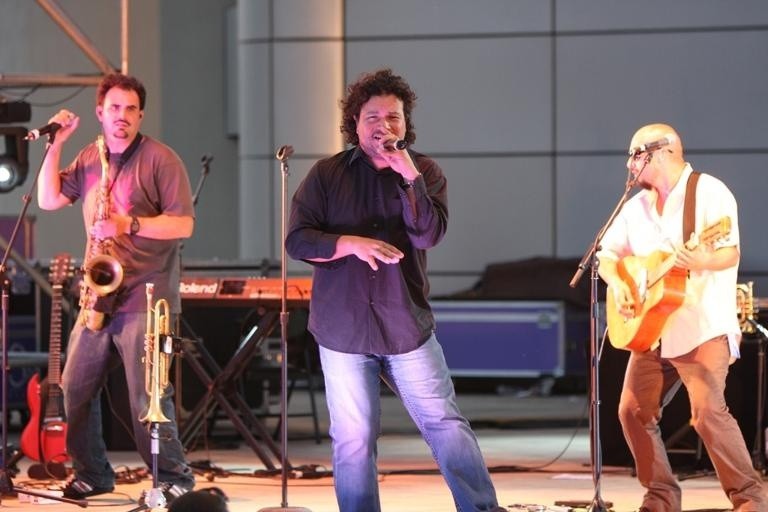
[[0, 127, 29, 193]]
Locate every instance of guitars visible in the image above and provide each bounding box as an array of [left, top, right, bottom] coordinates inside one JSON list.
[[605, 216, 732, 353], [19, 252, 74, 463]]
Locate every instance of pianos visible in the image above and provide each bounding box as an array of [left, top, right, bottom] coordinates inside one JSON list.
[[176, 273, 311, 304]]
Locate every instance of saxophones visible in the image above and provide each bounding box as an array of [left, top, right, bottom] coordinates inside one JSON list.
[[78, 135, 124, 330]]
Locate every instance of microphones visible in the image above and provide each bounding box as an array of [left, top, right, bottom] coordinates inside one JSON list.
[[24, 122, 61, 141], [384, 139, 407, 150], [627, 133, 675, 156]]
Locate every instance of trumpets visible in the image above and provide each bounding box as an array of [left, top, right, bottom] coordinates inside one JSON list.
[[139, 283, 172, 425]]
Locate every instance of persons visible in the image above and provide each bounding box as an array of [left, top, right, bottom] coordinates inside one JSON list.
[[36, 73, 196, 508], [596, 122, 768, 512], [285, 67, 510, 512]]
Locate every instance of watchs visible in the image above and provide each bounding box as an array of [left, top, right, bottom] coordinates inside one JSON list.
[[129, 213, 140, 237]]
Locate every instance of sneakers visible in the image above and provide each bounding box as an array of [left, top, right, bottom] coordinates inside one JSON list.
[[137, 479, 194, 508], [60, 476, 114, 500]]
